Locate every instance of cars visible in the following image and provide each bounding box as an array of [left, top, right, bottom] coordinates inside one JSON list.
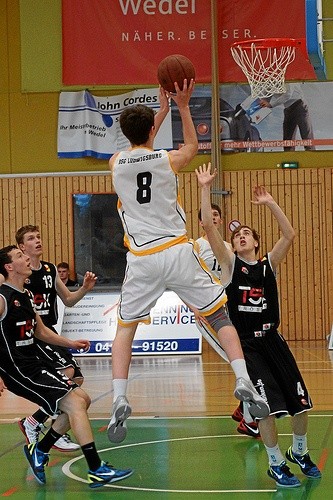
[[170, 97, 254, 157]]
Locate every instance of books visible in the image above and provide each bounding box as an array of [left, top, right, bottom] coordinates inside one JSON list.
[[241, 91, 272, 125]]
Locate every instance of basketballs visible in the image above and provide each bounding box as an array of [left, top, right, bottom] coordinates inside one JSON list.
[[158, 54, 195, 93]]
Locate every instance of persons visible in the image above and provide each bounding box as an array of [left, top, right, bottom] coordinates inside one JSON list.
[[56, 262, 77, 286], [260, 83, 316, 151], [0, 245, 135, 489], [0, 225, 98, 452], [195, 162, 322, 487], [177, 203, 262, 440], [107, 78, 270, 443]]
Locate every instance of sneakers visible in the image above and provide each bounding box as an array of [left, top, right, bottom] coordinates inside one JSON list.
[[16, 415, 82, 486], [231, 377, 270, 439], [106, 395, 132, 444], [267, 447, 323, 488], [87, 462, 136, 488]]
